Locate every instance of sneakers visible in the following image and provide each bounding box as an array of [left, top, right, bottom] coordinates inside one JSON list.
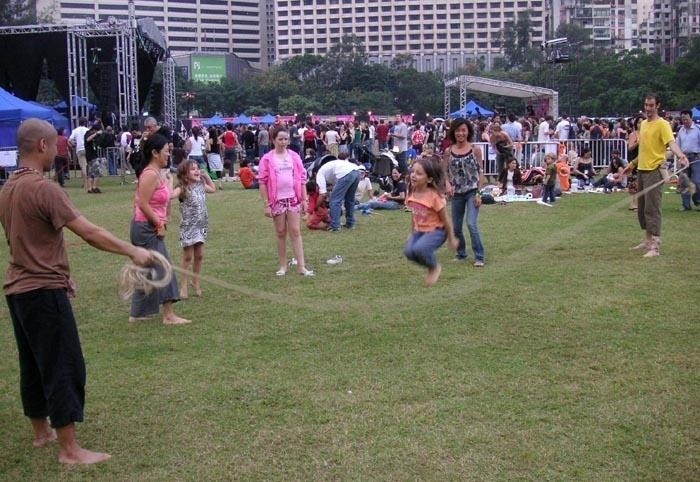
[[342, 224, 355, 232], [275, 267, 289, 277], [287, 258, 297, 267], [88, 190, 94, 193], [326, 255, 343, 265], [326, 226, 341, 233], [92, 187, 101, 193], [296, 269, 316, 279]]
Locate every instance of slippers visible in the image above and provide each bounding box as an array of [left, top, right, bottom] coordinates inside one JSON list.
[[629, 208, 638, 212]]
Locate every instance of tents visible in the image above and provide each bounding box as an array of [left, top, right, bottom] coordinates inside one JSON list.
[[450, 103, 494, 119], [206, 114, 276, 127]]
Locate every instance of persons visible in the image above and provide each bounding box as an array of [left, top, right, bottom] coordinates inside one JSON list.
[[56, 116, 172, 193], [288, 114, 450, 231], [257, 129, 309, 275], [469, 106, 647, 203], [129, 136, 217, 324], [405, 118, 484, 284], [171, 123, 285, 189], [621, 95, 689, 257], [660, 110, 699, 209], [1, 117, 160, 466]]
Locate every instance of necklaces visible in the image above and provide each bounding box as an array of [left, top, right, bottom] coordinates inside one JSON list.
[[14, 167, 46, 178]]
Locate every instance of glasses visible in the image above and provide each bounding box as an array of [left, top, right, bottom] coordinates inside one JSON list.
[[143, 126, 155, 130]]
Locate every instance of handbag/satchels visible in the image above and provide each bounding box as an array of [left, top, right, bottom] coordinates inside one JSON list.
[[532, 186, 543, 198], [627, 143, 638, 161]]
